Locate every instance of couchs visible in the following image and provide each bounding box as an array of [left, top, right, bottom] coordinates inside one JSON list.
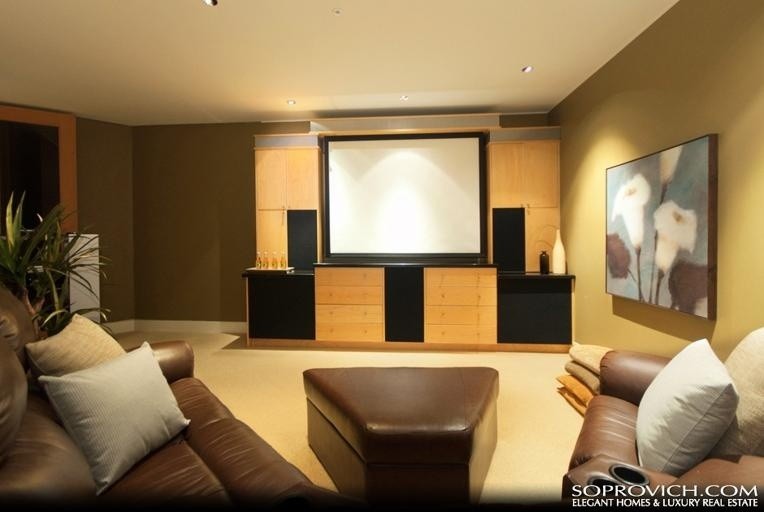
[[0, 286, 374, 512]]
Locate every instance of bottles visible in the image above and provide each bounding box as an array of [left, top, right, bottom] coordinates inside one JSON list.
[[539, 250, 550, 274], [255, 251, 262, 269], [271, 251, 279, 269], [261, 251, 269, 269], [280, 251, 287, 269]]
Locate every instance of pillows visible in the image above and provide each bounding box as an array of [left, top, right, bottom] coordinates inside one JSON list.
[[555, 345, 616, 414], [25, 313, 193, 495], [636, 327, 763, 477]]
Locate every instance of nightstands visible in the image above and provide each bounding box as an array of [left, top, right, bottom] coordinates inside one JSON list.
[[302, 366, 498, 509]]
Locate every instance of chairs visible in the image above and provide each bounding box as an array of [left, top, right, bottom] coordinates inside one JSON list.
[[561, 348, 763, 506]]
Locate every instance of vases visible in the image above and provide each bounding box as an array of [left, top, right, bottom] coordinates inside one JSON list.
[[539, 228, 566, 275]]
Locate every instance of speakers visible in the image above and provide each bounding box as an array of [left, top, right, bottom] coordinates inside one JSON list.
[[286, 208, 318, 274], [385, 267, 423, 341], [492, 206, 526, 274]]
